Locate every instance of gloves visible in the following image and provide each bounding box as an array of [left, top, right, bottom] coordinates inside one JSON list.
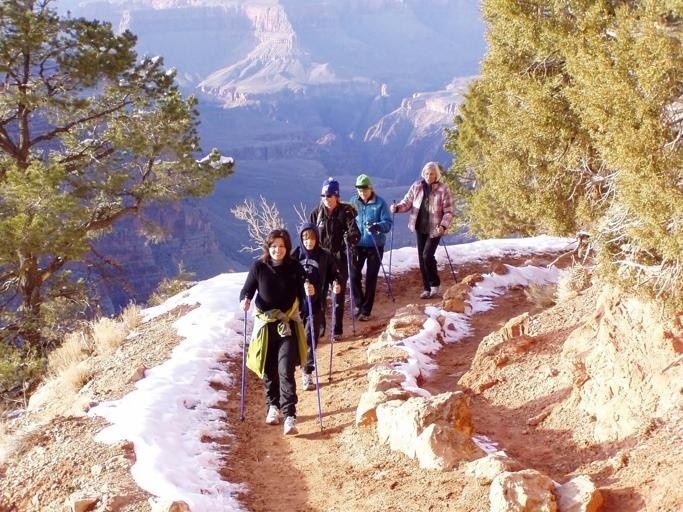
[[344, 233, 355, 244], [367, 223, 378, 234]]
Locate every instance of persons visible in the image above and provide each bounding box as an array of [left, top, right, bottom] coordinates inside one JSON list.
[[307, 177, 361, 338], [390, 161, 455, 298], [348, 173, 393, 321], [239, 228, 315, 435], [291, 223, 344, 392]]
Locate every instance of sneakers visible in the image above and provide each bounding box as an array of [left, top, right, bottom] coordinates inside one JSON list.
[[421, 290, 430, 298], [358, 314, 369, 321], [266, 406, 279, 424], [333, 335, 342, 341], [430, 287, 439, 297], [353, 307, 360, 315], [284, 416, 299, 435], [302, 375, 316, 391]]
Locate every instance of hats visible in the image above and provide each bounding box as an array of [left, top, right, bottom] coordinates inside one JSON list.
[[321, 178, 340, 198], [355, 174, 372, 191]]
